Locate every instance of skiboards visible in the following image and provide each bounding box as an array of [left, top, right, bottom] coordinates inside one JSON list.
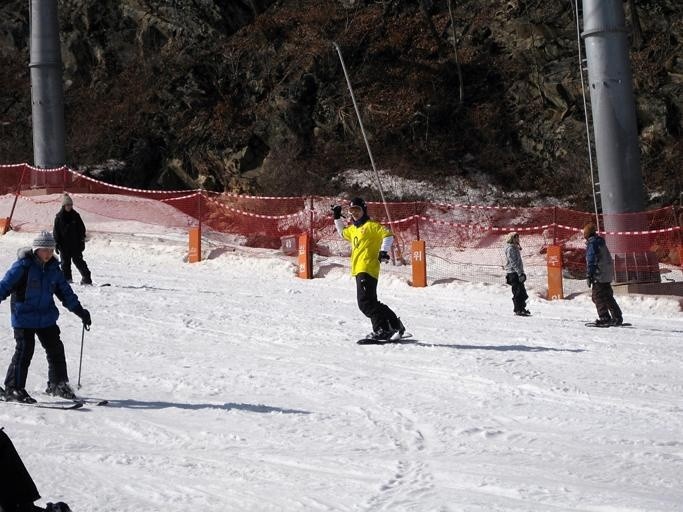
[[0, 386, 108, 410], [357, 331, 417, 343], [585, 319, 632, 327]]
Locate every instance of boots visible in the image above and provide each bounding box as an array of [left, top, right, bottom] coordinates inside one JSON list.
[[79, 272, 92, 284]]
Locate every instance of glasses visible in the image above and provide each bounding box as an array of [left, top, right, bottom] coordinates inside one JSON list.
[[348, 206, 362, 215]]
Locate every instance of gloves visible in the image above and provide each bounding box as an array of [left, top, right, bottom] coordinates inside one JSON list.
[[79, 241, 85, 251], [377, 251, 390, 264], [333, 205, 343, 219], [78, 307, 92, 326], [55, 244, 60, 255]]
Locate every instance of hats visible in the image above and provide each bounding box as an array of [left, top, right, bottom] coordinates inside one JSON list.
[[506, 231, 520, 244], [31, 229, 55, 256], [61, 192, 74, 206], [583, 223, 597, 237]]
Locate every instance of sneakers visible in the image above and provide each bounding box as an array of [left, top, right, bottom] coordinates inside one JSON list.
[[45, 380, 77, 400], [4, 385, 37, 404], [366, 328, 402, 340]]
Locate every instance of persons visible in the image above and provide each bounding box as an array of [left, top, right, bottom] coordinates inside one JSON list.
[[584, 221, 623, 328], [504, 231, 533, 317], [53, 193, 92, 285], [0, 427, 73, 512], [0, 231, 91, 404], [331, 197, 413, 344]]
